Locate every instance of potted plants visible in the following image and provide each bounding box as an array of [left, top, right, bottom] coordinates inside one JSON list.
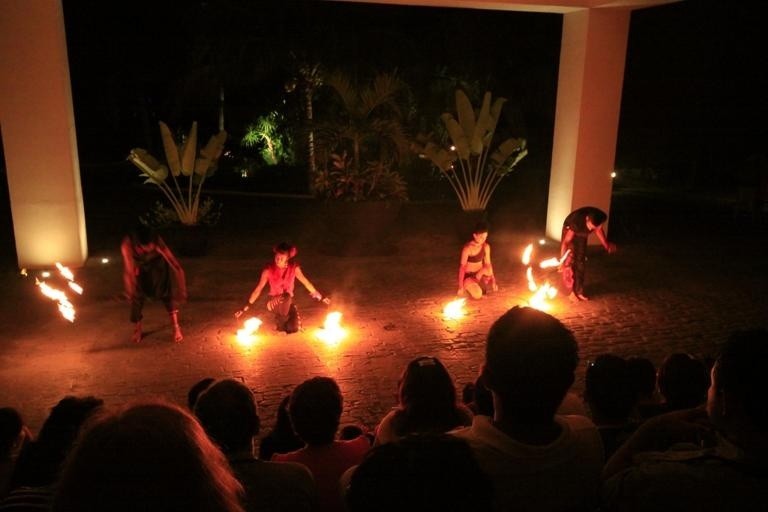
[[299, 67, 424, 261], [410, 77, 532, 229], [122, 118, 231, 258]]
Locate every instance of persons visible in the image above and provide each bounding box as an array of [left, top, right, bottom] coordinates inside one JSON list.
[[233, 243, 331, 333], [457, 224, 498, 298], [120, 226, 187, 343], [0, 305, 766, 512], [560, 207, 617, 303]]
